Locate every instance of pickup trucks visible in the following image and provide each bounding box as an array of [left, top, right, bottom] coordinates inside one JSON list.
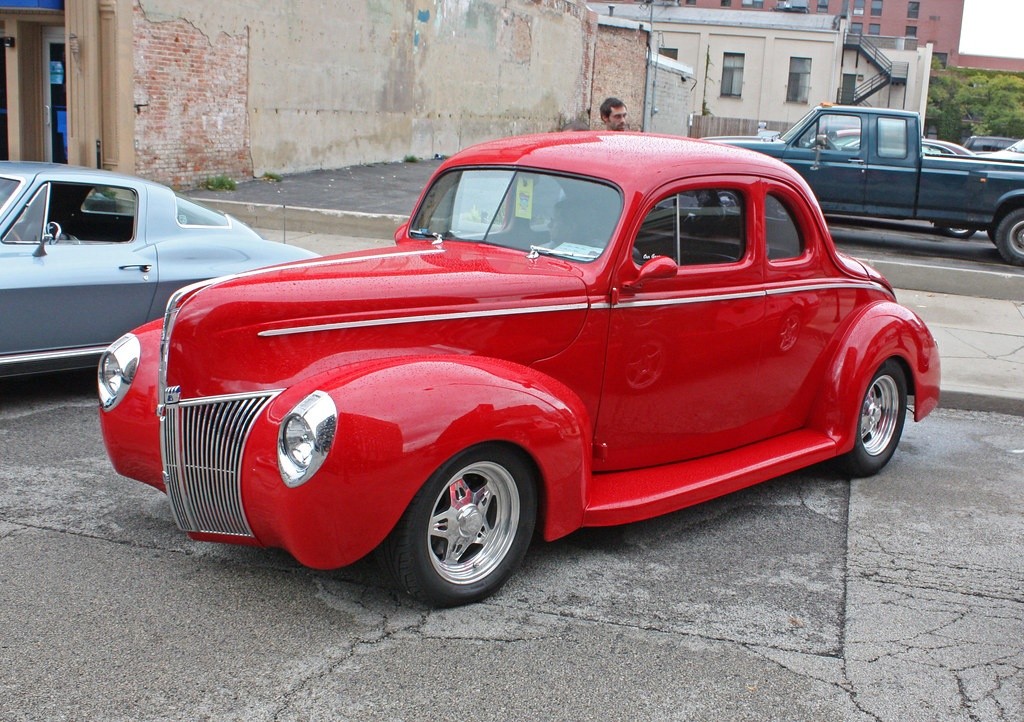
[[697, 103, 1024, 268]]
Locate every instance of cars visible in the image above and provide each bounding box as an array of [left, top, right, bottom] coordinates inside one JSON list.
[[0, 159, 322, 380], [96, 128, 942, 611], [811, 127, 1024, 241]]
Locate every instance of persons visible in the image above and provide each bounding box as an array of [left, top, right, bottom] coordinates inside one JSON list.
[[602, 99, 628, 134], [536, 201, 605, 254]]
